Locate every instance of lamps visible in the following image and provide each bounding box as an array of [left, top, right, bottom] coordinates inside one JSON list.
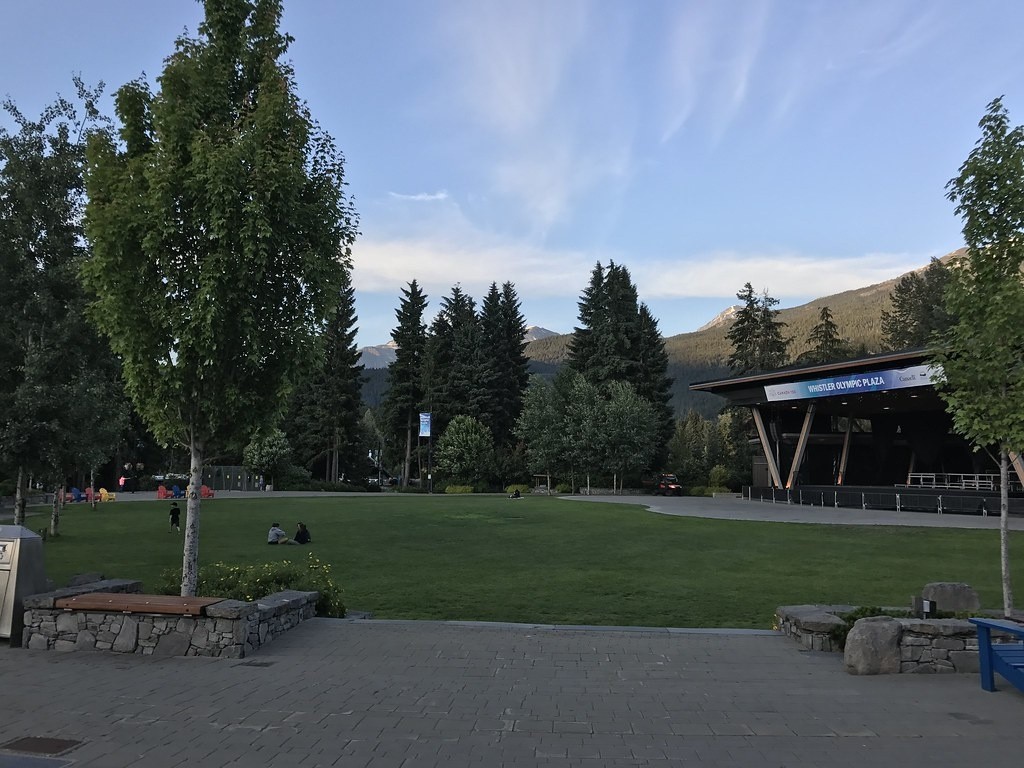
[[923, 599, 937, 615]]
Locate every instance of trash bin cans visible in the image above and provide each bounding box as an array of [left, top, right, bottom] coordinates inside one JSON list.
[[0, 523, 43, 641]]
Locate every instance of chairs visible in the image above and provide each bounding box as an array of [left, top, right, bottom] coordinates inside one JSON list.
[[201, 485, 214, 499], [157, 485, 173, 499], [99, 488, 115, 503], [85, 487, 102, 502], [967, 617, 1024, 695], [59, 489, 73, 503], [185, 485, 190, 497], [71, 488, 88, 503], [171, 485, 185, 498]]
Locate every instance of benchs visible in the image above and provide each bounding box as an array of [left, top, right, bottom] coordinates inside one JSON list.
[[57, 591, 227, 618]]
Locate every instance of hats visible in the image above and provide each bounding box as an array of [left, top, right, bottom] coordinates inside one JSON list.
[[170, 502, 177, 505]]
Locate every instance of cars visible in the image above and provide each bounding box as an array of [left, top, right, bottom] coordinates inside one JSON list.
[[652, 474, 683, 497], [337, 472, 422, 487]]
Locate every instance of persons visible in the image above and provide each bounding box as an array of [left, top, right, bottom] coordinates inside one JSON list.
[[119, 476, 126, 493], [509, 489, 520, 498], [168, 502, 182, 535], [268, 522, 289, 545], [287, 521, 311, 545]]
